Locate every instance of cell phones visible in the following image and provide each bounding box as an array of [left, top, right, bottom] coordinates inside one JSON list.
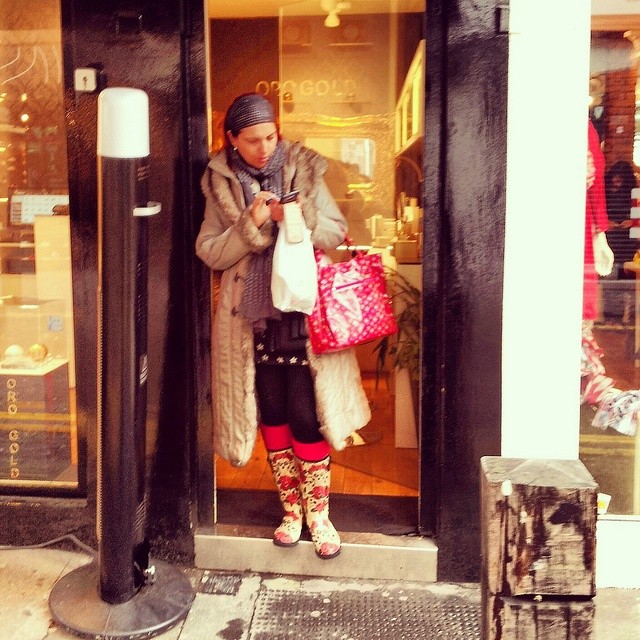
[[277, 189, 300, 203]]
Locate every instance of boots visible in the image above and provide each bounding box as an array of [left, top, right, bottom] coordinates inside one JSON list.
[[266, 449, 304, 549], [290, 455, 342, 559]]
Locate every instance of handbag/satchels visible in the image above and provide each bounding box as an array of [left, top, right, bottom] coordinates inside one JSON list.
[[305, 221, 399, 352], [393, 361, 417, 450]]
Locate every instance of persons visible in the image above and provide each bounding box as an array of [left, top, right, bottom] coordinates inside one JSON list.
[[604, 161, 638, 280], [193, 93, 373, 559], [580, 96, 614, 361]]
